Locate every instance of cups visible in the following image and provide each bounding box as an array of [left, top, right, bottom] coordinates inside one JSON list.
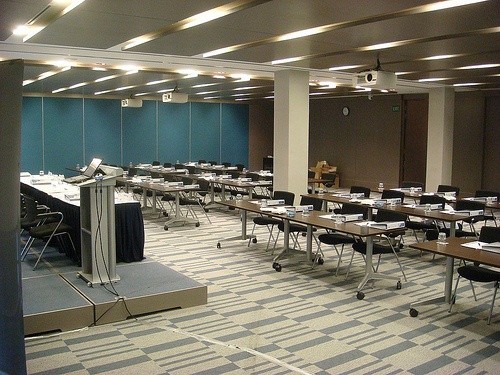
[[238, 178, 241, 182], [165, 181, 168, 186], [379, 183, 383, 188], [150, 179, 153, 183], [262, 199, 267, 206], [488, 197, 492, 204], [237, 194, 242, 200], [40, 171, 44, 175], [318, 190, 323, 194], [336, 215, 342, 223], [426, 204, 431, 211], [439, 232, 445, 241], [243, 168, 246, 172], [352, 195, 357, 199], [303, 209, 309, 216], [411, 187, 414, 193]]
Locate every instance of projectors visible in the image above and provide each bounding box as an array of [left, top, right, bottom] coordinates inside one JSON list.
[[120, 98, 143, 108], [353, 70, 396, 88], [162, 93, 189, 103]]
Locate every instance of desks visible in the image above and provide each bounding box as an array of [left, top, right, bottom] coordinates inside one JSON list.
[[175, 172, 230, 210], [65, 165, 85, 175], [249, 169, 273, 181], [451, 195, 500, 232], [181, 160, 213, 174], [137, 182, 201, 230], [147, 166, 187, 202], [203, 165, 240, 176], [389, 185, 466, 232], [203, 178, 273, 221], [214, 199, 321, 269], [409, 237, 500, 318], [115, 175, 167, 216], [275, 211, 409, 301], [19, 173, 145, 266], [368, 201, 473, 305], [130, 164, 163, 195], [298, 190, 408, 244], [308, 176, 334, 195]]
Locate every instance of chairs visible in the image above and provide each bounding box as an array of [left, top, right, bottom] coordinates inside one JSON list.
[[20, 159, 500, 328]]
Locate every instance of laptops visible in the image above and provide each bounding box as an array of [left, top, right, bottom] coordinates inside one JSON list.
[[64, 157, 103, 183]]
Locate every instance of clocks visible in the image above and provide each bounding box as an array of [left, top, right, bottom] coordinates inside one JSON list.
[[341, 104, 350, 117]]
